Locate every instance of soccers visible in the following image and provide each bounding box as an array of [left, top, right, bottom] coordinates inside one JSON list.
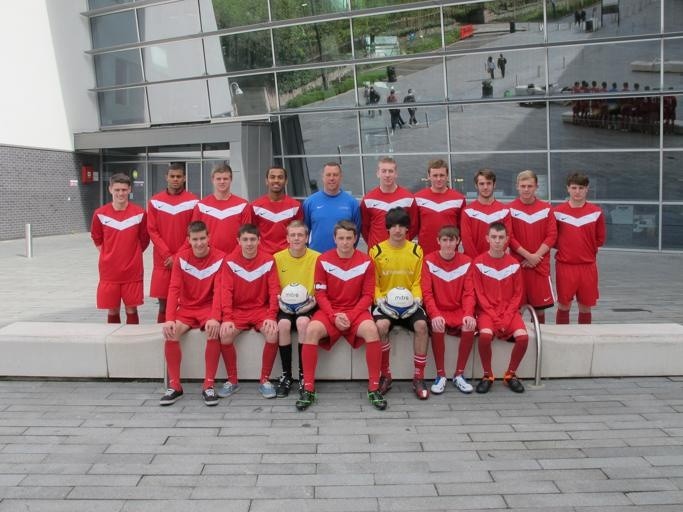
[[386, 285, 414, 321]]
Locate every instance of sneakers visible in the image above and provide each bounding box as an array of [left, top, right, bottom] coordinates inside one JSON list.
[[295, 390, 318, 410], [259, 381, 276, 398], [412, 378, 429, 399], [298, 371, 305, 395], [159, 388, 184, 405], [276, 372, 293, 397], [380, 373, 392, 394], [475, 374, 493, 393], [201, 387, 219, 405], [430, 375, 447, 394], [504, 371, 524, 392], [366, 389, 387, 411], [216, 381, 240, 397], [452, 374, 473, 393]]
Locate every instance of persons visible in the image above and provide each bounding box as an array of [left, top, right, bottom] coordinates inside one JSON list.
[[608, 81, 619, 129], [361, 156, 419, 254], [389, 96, 407, 129], [363, 86, 373, 116], [631, 83, 641, 132], [472, 222, 529, 394], [668, 86, 678, 134], [218, 224, 279, 400], [193, 164, 251, 253], [370, 86, 382, 118], [620, 82, 631, 132], [589, 80, 600, 127], [579, 80, 589, 128], [387, 66, 398, 81], [368, 207, 430, 400], [462, 168, 512, 259], [554, 173, 606, 323], [497, 54, 507, 78], [241, 167, 303, 257], [91, 173, 150, 324], [159, 222, 224, 406], [422, 224, 476, 394], [387, 90, 399, 131], [574, 9, 580, 27], [404, 89, 420, 127], [273, 220, 324, 398], [301, 163, 362, 253], [664, 96, 670, 134], [580, 9, 587, 25], [652, 87, 660, 135], [147, 164, 200, 324], [487, 56, 495, 80], [506, 169, 558, 324], [572, 81, 580, 127], [413, 158, 467, 256], [295, 222, 388, 411], [601, 81, 608, 128], [641, 85, 651, 133]]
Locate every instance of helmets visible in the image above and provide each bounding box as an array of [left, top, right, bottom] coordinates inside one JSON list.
[[384, 286, 414, 316]]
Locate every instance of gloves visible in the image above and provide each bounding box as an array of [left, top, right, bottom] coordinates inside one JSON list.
[[376, 299, 399, 320], [276, 294, 294, 314], [400, 301, 419, 319], [296, 296, 316, 314]]
